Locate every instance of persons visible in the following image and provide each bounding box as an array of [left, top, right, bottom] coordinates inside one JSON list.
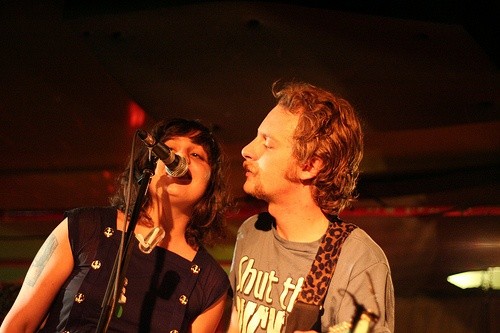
[[0, 117, 232, 333], [217, 84, 396, 333]]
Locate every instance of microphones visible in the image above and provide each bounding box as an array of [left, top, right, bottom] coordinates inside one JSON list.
[[138, 130, 189, 178]]
